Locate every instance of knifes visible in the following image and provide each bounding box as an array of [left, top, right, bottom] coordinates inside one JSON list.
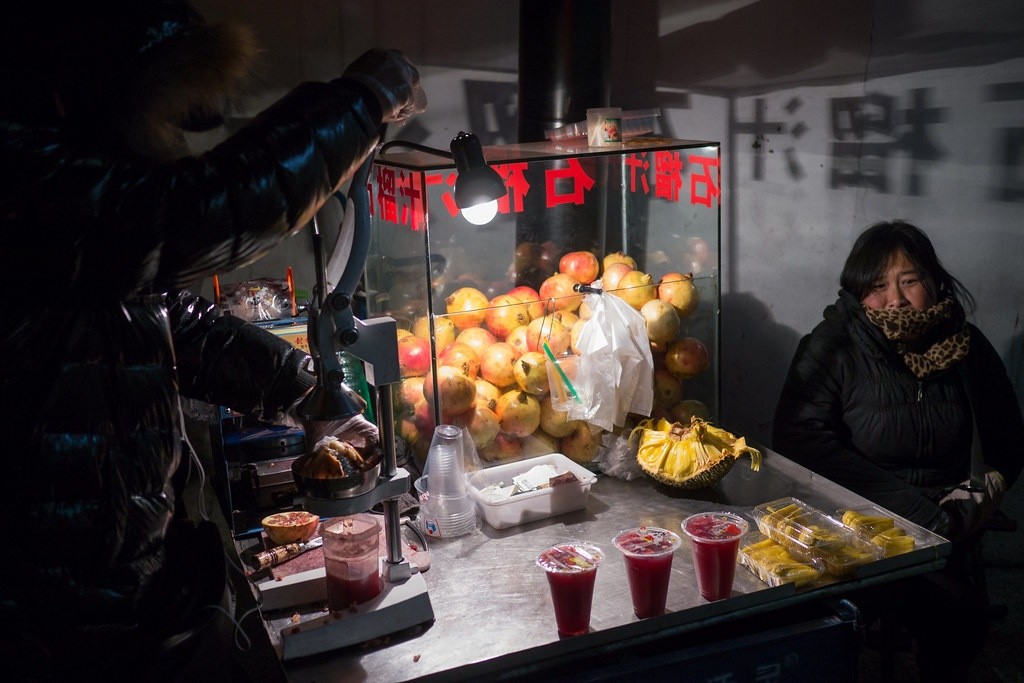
[[253, 537, 323, 564]]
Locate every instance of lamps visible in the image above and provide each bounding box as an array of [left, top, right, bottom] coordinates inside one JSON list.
[[381, 128, 508, 225]]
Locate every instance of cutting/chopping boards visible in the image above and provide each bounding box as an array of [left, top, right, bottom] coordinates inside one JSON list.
[[260, 517, 428, 577]]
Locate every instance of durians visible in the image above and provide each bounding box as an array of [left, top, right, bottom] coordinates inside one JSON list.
[[636, 417, 761, 488]]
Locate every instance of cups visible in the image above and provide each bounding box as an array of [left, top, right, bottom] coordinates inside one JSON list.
[[416, 426, 476, 538], [538, 544, 603, 635], [614, 528, 682, 618], [681, 512, 749, 600], [320, 516, 379, 605], [546, 355, 584, 412]]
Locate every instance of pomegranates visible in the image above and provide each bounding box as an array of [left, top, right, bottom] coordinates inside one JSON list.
[[309, 440, 365, 478], [262, 512, 320, 544], [393, 254, 707, 460]]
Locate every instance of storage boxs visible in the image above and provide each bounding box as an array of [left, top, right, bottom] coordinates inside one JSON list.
[[462, 452, 598, 531]]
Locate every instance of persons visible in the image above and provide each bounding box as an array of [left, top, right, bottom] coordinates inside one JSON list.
[[773, 222, 1024, 683], [0, 0, 416, 683]]
